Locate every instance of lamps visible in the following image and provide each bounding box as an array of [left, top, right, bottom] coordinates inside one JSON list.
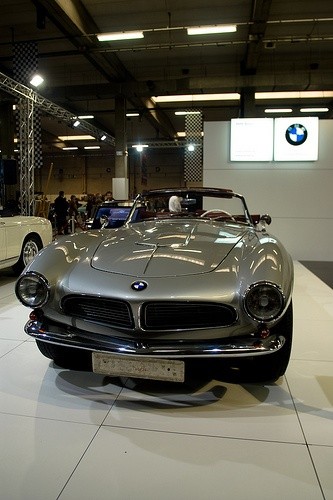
[[254, 89, 333, 100], [186, 24, 237, 35], [153, 93, 241, 102], [97, 30, 144, 42]]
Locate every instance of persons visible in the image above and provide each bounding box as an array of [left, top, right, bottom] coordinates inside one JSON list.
[[86, 193, 96, 218], [169, 196, 181, 212], [106, 192, 118, 207], [54, 191, 70, 235], [69, 195, 78, 232], [93, 198, 103, 218]]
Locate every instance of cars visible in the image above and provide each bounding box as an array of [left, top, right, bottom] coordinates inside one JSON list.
[[13, 188, 296, 388], [0, 208, 53, 276]]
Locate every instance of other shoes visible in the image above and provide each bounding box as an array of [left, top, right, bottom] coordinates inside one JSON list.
[[64, 232, 70, 235], [58, 232, 63, 235]]
